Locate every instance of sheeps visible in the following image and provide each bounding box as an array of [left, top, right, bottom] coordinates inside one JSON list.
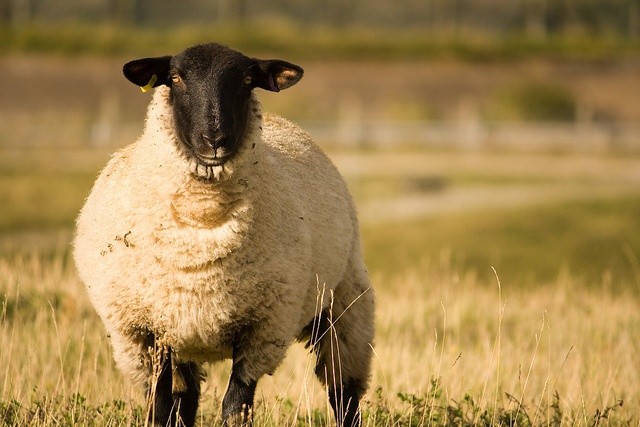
[[72, 41, 374, 426]]
